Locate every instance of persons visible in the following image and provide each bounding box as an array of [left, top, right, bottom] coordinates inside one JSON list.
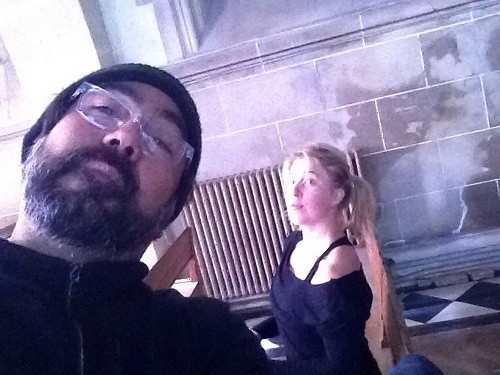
[[271, 142, 444, 375], [0, 63, 274, 375]]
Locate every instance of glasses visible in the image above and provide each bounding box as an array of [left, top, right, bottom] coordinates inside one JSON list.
[[67, 82, 194, 167]]
